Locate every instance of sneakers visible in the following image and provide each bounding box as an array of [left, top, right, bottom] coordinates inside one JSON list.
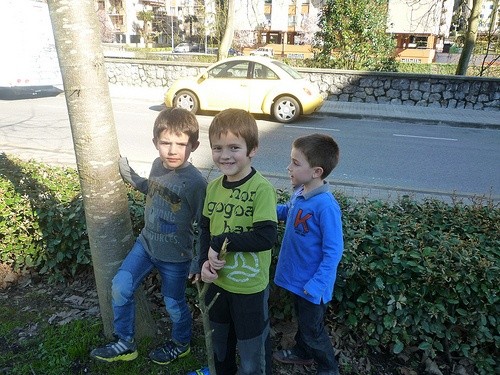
[[273, 348, 314, 364], [89, 333, 138, 362], [188, 366, 211, 375], [149, 339, 190, 364]]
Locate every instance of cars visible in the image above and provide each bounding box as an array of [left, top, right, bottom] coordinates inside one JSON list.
[[164, 55, 325, 124], [249, 47, 273, 57], [172, 42, 244, 57]]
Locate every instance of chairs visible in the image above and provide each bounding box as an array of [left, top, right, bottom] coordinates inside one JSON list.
[[255, 69, 262, 78]]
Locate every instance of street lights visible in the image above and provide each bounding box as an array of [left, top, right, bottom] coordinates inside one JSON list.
[[184, 15, 199, 44]]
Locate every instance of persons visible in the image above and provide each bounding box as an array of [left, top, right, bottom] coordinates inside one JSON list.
[[90, 107, 207, 365], [272, 134, 344, 375], [187, 108, 278, 375]]
